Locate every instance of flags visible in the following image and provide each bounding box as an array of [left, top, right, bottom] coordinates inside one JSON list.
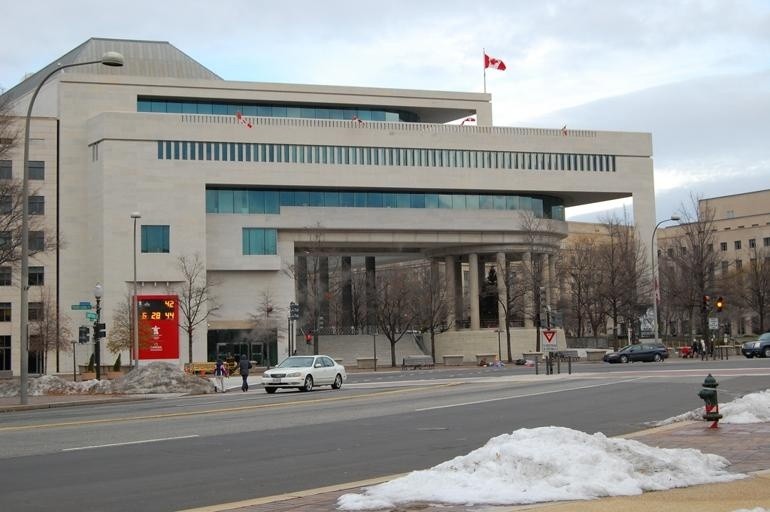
[[237, 111, 252, 129], [461, 118, 475, 125], [562, 128, 567, 136], [485, 55, 506, 70], [352, 116, 366, 126]]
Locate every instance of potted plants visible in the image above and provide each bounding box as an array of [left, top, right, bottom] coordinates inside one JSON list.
[[80, 353, 125, 381]]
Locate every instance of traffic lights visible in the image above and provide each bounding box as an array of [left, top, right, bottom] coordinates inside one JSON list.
[[319, 317, 324, 329], [714, 297, 723, 312], [703, 295, 710, 312], [290, 302, 299, 320]]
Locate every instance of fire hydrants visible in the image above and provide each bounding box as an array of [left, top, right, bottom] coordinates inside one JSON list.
[[697, 374, 722, 427]]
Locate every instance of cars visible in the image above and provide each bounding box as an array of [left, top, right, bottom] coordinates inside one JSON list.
[[741, 333, 770, 358], [603, 343, 668, 363], [261, 355, 346, 394]]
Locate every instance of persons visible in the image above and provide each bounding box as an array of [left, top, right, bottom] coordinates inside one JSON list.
[[682, 336, 714, 358], [216, 357, 222, 375], [239, 354, 252, 392]]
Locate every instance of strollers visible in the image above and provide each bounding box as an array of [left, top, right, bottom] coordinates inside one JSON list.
[[682, 347, 693, 358]]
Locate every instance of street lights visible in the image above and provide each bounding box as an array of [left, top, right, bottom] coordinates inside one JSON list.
[[372, 333, 378, 371], [19, 50, 124, 403], [650, 217, 681, 343], [129, 212, 141, 370], [495, 329, 504, 363]]
